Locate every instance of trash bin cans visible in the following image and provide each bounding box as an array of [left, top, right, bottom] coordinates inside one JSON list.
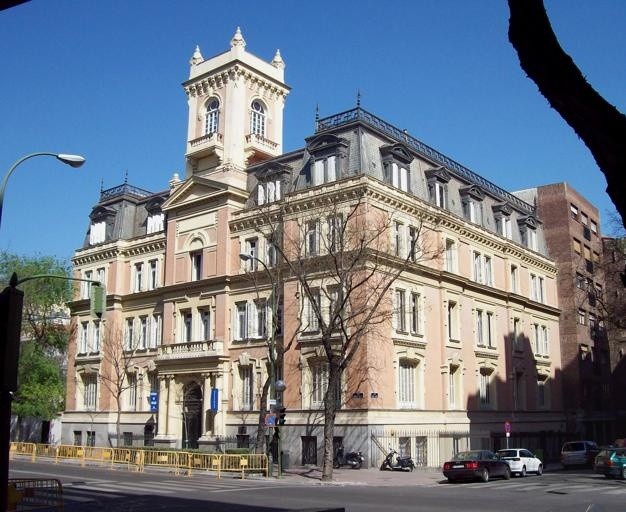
[[280, 450, 290, 469], [260, 452, 273, 476]]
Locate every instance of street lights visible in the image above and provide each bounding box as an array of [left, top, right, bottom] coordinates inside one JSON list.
[[0, 151, 86, 231], [238, 252, 276, 477]]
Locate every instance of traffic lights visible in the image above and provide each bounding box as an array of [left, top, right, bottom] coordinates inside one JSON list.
[[279, 406, 286, 425]]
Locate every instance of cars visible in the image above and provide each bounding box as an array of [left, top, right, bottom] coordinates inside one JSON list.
[[442, 450, 511, 482], [495, 448, 544, 477], [560, 441, 626, 480]]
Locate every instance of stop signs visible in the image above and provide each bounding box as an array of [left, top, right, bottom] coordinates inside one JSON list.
[[505, 422, 511, 432]]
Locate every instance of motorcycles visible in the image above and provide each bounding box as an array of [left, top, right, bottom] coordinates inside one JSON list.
[[379, 447, 416, 472], [332, 444, 365, 469]]
